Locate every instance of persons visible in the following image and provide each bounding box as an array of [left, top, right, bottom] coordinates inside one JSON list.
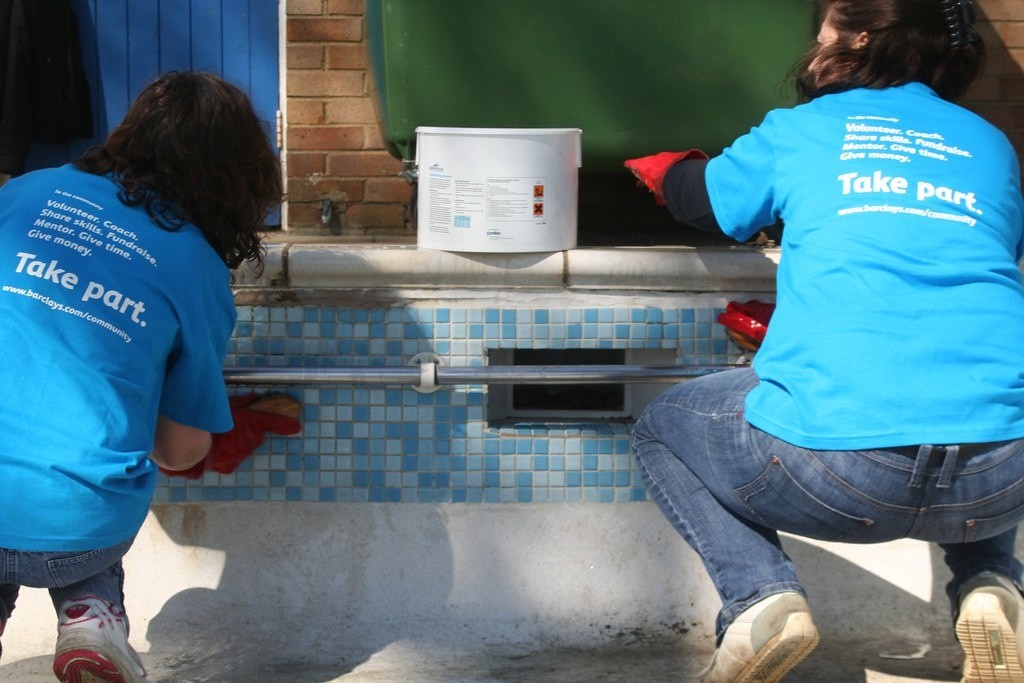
[[622, 1, 1024, 683], [1, 71, 300, 683]]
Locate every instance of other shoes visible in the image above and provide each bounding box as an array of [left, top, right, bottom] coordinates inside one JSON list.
[[954, 569, 1023, 683], [697, 589, 821, 683]]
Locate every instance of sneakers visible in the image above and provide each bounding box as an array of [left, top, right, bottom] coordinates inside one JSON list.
[[53, 593, 153, 683]]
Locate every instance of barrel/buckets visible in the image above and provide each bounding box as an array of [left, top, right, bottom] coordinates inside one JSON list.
[[416, 126, 582, 253]]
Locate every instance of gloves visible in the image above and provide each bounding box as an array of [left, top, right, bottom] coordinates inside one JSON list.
[[717, 299, 775, 342], [158, 393, 301, 480], [622, 148, 710, 207]]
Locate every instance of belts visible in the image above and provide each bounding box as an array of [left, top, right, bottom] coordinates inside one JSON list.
[[891, 445, 1001, 466]]
